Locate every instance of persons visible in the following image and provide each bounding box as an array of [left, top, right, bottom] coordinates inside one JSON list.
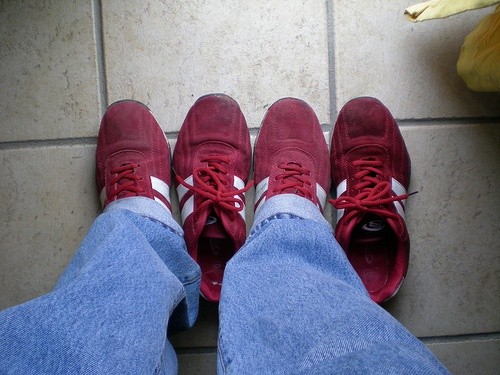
[[0, 98, 453, 375]]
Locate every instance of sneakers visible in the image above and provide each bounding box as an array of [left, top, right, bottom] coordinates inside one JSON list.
[[331, 97, 411, 307], [174, 94, 253, 301], [96, 99, 174, 215], [252, 98, 332, 215]]
